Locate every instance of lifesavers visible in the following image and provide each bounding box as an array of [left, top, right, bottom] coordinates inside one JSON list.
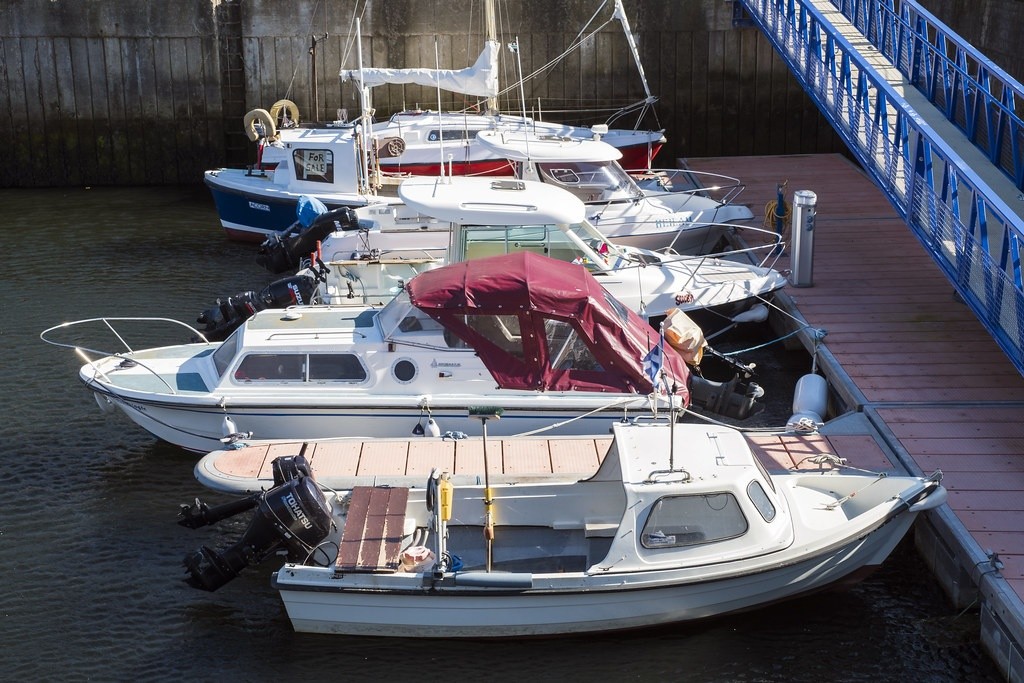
[[269, 99, 300, 128], [243, 108, 276, 142]]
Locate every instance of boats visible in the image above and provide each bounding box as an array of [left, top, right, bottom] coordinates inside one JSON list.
[[168, 321, 946, 640], [40, 252, 830, 455], [204, 0, 756, 255], [190, 33, 794, 345]]
